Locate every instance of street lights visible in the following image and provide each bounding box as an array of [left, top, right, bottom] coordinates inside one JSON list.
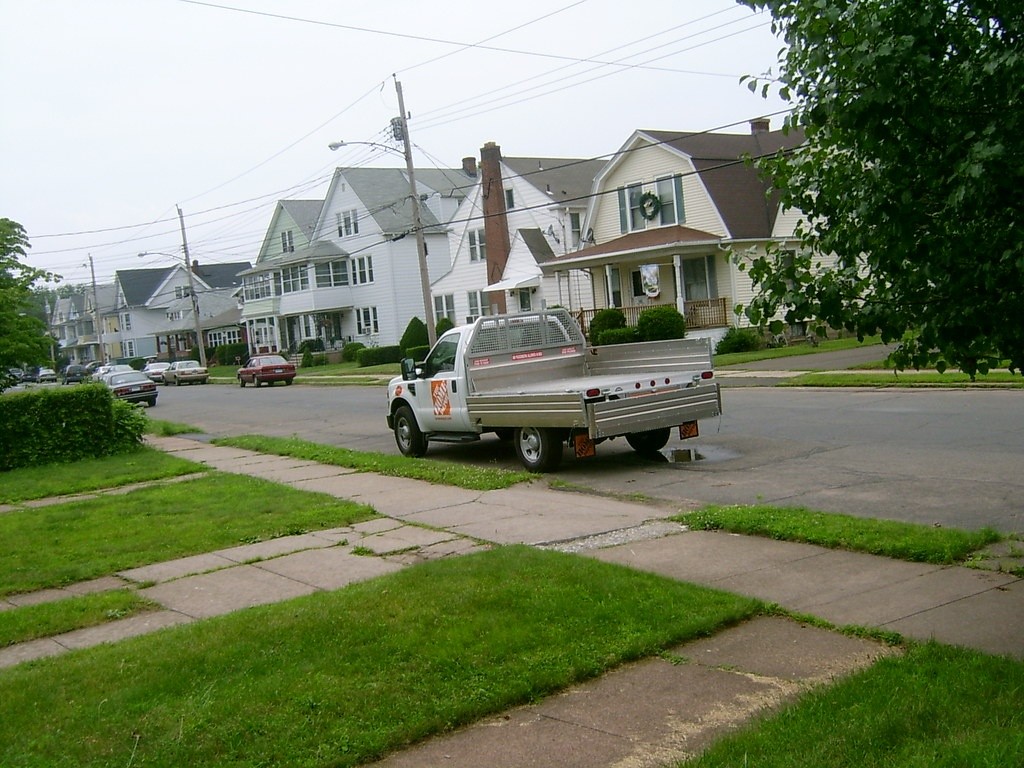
[[138, 251, 208, 369], [329, 140, 438, 354]]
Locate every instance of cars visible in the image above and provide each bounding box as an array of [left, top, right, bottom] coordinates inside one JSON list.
[[105, 370, 159, 409], [161, 360, 210, 386], [236, 355, 297, 387], [36, 368, 58, 384], [5, 368, 23, 383], [60, 359, 135, 390], [140, 363, 171, 383], [21, 372, 33, 383]]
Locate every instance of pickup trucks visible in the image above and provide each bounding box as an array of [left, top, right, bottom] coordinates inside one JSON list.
[[384, 308, 724, 471]]
